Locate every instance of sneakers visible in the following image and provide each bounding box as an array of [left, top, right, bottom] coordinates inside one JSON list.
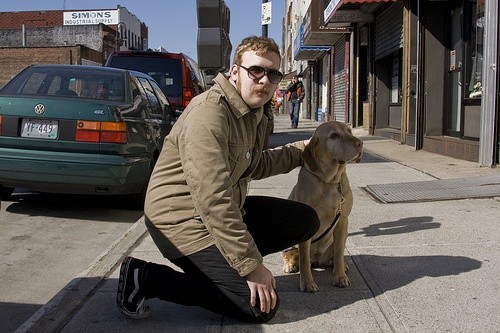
[[116, 256, 153, 320]]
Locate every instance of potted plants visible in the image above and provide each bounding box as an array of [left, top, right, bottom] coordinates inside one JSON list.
[[263, 94, 277, 134]]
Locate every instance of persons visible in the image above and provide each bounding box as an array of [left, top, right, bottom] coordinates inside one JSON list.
[[116, 36, 320, 325], [285, 76, 305, 129]]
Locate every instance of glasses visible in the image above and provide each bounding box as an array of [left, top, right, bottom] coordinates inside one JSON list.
[[239, 64, 284, 84]]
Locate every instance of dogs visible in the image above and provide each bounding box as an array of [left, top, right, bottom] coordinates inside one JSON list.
[[283, 122, 363, 293]]
